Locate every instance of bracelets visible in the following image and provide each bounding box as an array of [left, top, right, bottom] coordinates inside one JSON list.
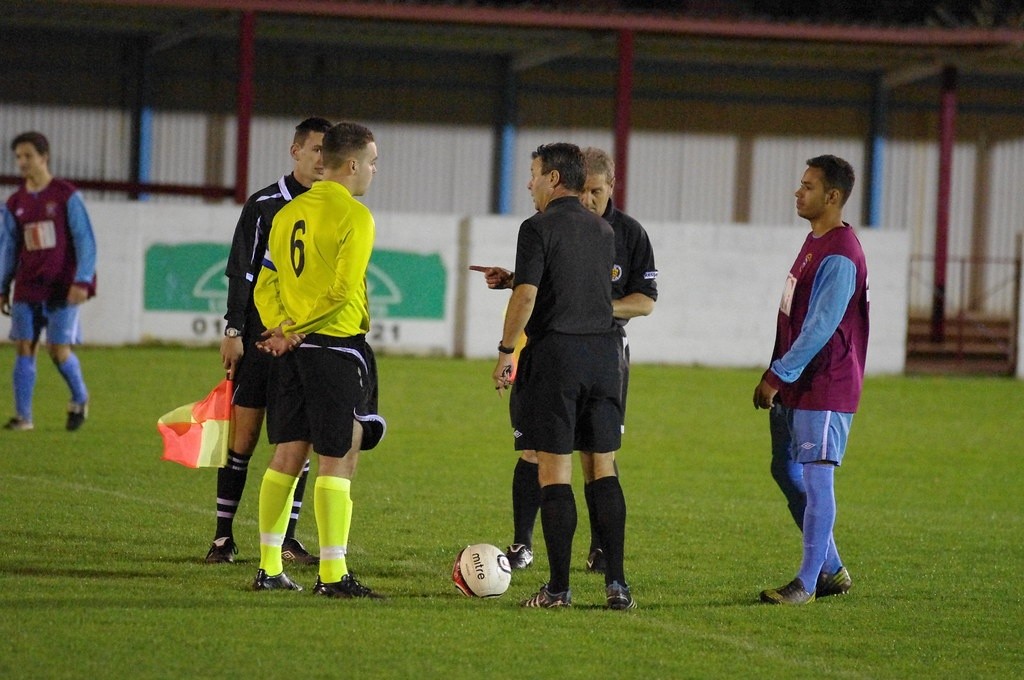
[[498, 341, 515, 354]]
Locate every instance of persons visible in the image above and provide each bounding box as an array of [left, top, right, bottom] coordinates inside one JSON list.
[[256, 122, 395, 599], [206, 119, 333, 569], [0, 132, 96, 432], [469, 146, 657, 572], [493, 143, 639, 611], [753, 154, 871, 606]]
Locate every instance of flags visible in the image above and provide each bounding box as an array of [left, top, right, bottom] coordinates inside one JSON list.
[[157, 379, 233, 469]]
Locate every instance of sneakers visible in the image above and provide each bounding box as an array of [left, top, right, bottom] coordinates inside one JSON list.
[[759, 577, 816, 604], [251, 569, 304, 592], [205, 537, 238, 563], [66, 395, 89, 431], [5, 415, 34, 430], [605, 581, 638, 609], [519, 583, 573, 608], [586, 548, 606, 571], [815, 566, 852, 598], [281, 538, 321, 565], [312, 568, 386, 599], [506, 544, 534, 569]]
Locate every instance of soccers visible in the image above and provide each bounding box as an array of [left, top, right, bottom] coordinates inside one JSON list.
[[451, 543, 512, 600]]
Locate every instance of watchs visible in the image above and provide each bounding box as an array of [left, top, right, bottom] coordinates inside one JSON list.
[[224, 328, 244, 338]]
